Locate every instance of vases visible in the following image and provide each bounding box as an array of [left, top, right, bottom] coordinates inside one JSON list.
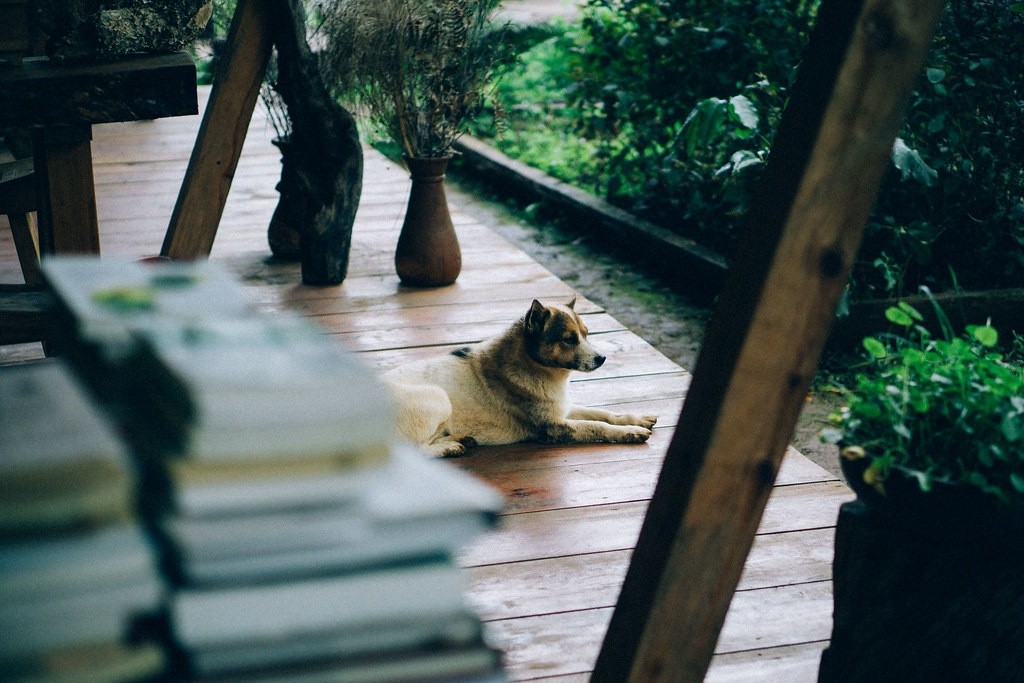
[[394, 152, 462, 287], [268, 136, 300, 263]]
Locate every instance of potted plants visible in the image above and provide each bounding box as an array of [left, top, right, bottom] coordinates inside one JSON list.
[[815, 282, 1024, 529]]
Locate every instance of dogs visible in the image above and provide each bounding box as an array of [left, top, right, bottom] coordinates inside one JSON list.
[[376, 297, 657, 457]]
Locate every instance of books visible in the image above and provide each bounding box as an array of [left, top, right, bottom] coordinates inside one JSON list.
[[0, 258, 506, 683]]
